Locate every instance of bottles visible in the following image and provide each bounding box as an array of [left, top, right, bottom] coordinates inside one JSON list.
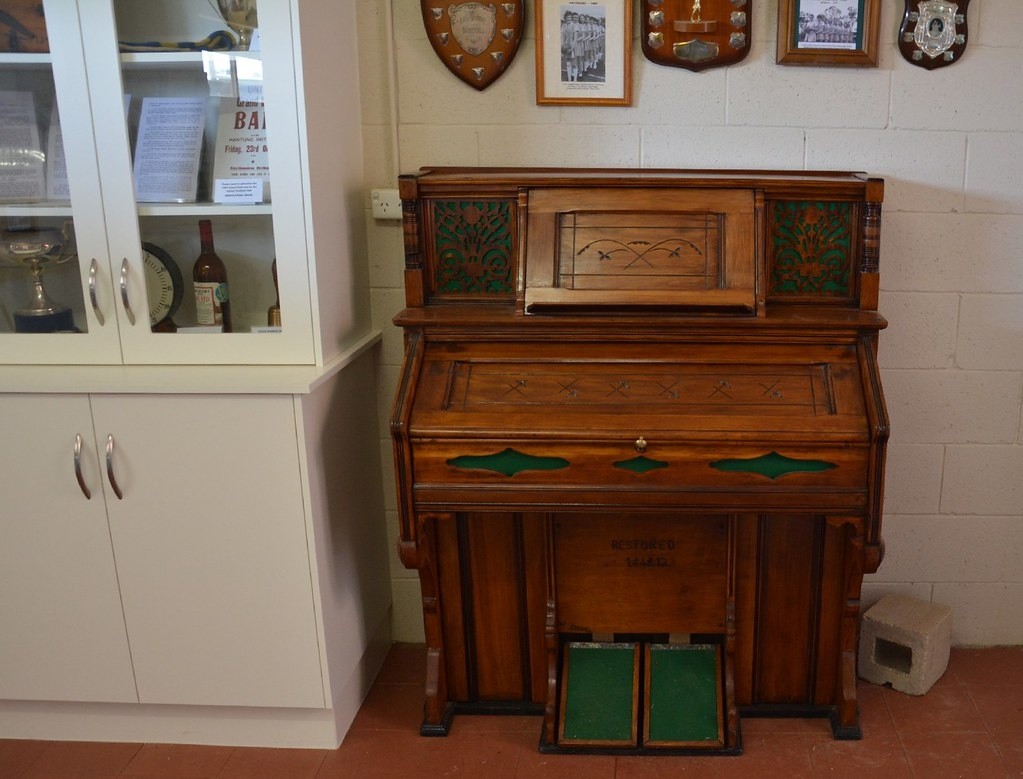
[[192, 217, 232, 333]]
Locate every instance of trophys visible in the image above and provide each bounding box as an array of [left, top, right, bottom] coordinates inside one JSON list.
[[216, 0, 257, 50], [0, 220, 78, 333]]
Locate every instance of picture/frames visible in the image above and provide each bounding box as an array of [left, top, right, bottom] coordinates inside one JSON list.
[[776, 0, 882, 68], [533, 0, 635, 108]]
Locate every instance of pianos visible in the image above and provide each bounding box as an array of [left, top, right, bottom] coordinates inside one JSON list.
[[389, 163, 891, 749]]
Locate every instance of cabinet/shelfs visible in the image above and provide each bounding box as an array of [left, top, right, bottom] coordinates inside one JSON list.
[[0, 0, 397, 752]]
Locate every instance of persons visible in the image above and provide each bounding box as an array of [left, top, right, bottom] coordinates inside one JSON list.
[[804, 14, 853, 42], [562, 11, 605, 82]]
